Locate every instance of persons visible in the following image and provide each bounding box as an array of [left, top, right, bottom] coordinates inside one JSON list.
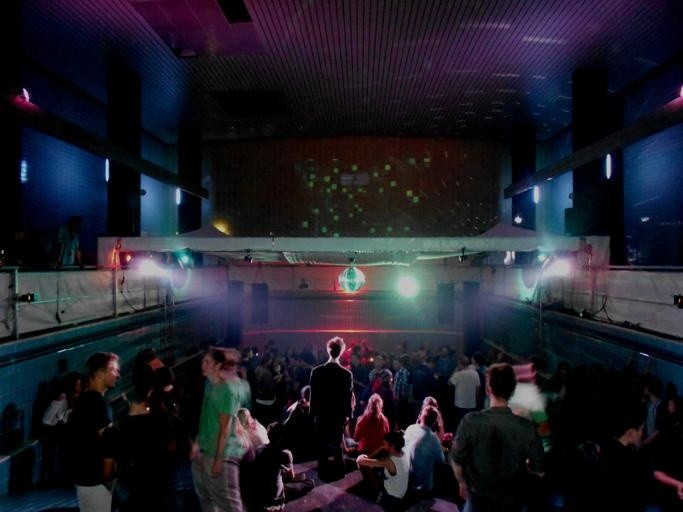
[[56, 215, 79, 265], [38, 336, 682, 512]]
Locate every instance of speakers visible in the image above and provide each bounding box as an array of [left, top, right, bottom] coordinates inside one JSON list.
[[174, 136, 202, 234], [571, 58, 628, 265], [509, 123, 538, 234], [107, 63, 142, 236]]
